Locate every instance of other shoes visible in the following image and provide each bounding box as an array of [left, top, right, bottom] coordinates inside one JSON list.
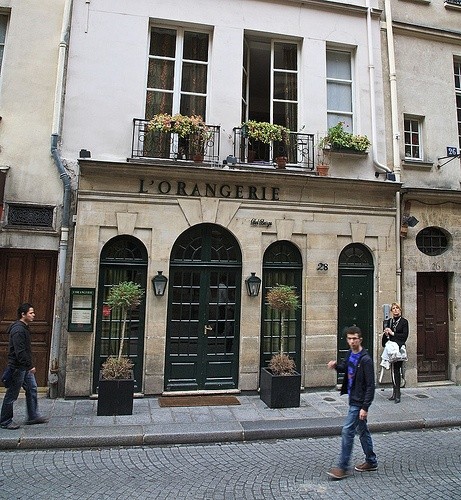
[[0, 422, 20, 430], [355, 461, 378, 471], [325, 468, 348, 478], [25, 415, 50, 424]]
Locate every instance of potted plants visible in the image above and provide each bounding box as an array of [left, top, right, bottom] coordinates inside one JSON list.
[[316, 136, 334, 176], [96, 279, 145, 417], [259, 282, 304, 410], [319, 114, 372, 156]]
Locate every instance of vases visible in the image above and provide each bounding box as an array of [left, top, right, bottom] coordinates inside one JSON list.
[[191, 152, 204, 163], [274, 156, 288, 169]]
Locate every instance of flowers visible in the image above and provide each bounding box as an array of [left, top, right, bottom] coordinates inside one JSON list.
[[145, 112, 214, 156]]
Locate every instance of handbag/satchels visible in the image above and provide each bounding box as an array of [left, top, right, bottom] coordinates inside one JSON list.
[[388, 345, 408, 361]]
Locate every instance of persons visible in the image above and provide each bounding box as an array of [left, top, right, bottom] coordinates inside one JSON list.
[[327, 326, 378, 477], [0, 303, 49, 429], [382, 303, 409, 403]]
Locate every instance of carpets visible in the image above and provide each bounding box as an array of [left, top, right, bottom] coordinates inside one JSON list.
[[158, 396, 242, 408]]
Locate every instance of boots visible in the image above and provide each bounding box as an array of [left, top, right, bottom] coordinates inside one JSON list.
[[388, 390, 396, 400], [395, 392, 400, 404]]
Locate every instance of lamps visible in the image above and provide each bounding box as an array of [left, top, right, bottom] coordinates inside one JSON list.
[[244, 272, 262, 297], [374, 171, 397, 182], [402, 215, 420, 227], [151, 270, 168, 297], [80, 148, 92, 159]]
